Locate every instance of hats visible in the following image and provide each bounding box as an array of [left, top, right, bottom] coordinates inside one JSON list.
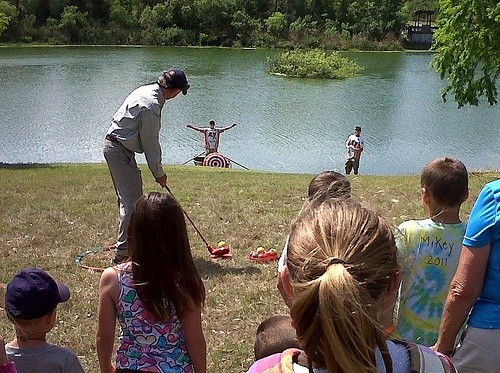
[[4, 269, 71, 319], [167, 67, 190, 95], [355, 126, 361, 131]]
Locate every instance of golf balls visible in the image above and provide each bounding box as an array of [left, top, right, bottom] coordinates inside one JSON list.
[[218, 240, 226, 248], [250, 246, 278, 258]]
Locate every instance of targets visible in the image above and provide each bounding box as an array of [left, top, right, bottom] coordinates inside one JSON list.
[[202, 152, 227, 168]]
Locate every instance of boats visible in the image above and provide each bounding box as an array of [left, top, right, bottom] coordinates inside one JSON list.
[[194, 156, 232, 168]]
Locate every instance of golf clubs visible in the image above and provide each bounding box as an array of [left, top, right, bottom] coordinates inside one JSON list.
[[164, 184, 230, 255]]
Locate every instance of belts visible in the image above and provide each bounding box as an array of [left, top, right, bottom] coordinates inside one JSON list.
[[105, 134, 119, 143]]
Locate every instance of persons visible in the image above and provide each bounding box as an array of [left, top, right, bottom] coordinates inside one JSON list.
[[245, 171, 460, 373], [5, 268, 86, 373], [429, 178, 500, 373], [345, 126, 364, 175], [377, 158, 470, 357], [102, 70, 190, 264], [95, 191, 207, 373], [187, 120, 237, 157]]
